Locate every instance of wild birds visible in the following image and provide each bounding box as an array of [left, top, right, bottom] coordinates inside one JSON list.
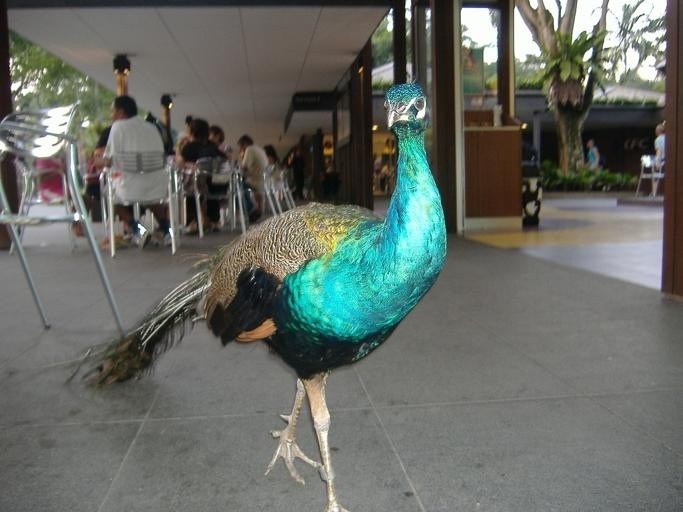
[[64, 81, 448, 512]]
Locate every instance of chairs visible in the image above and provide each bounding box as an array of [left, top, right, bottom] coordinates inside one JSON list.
[[100, 151, 297, 258], [1, 102, 126, 341], [635, 154, 664, 199]]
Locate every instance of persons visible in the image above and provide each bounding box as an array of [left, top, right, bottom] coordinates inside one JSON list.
[[650, 123, 664, 199], [93, 96, 174, 248], [84, 115, 311, 233], [584, 138, 601, 172]]
